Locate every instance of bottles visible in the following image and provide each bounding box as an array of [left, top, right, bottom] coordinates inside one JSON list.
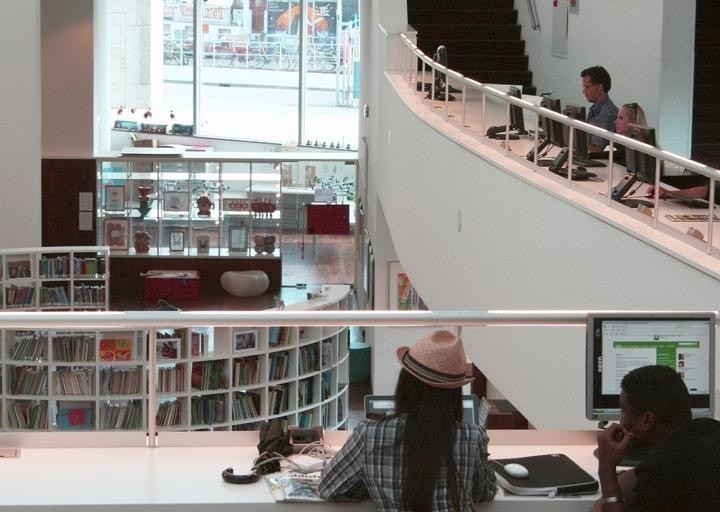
[[395, 330, 475, 390]]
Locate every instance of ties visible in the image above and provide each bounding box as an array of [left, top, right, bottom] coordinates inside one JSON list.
[[611, 123, 660, 208], [488, 86, 524, 140], [527, 97, 566, 167], [586, 311, 715, 466], [423, 45, 456, 101], [549, 103, 597, 180]]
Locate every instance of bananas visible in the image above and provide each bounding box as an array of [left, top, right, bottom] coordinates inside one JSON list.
[[566, 153, 607, 167], [529, 129, 546, 139], [661, 192, 716, 209]]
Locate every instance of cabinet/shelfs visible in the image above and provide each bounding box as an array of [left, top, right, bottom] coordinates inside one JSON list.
[[0, 245, 111, 311], [0, 284, 349, 432]]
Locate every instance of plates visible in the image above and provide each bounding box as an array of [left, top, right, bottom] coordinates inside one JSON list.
[[0, 245, 111, 311]]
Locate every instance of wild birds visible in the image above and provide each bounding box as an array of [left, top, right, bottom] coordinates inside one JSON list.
[[364, 396, 479, 427]]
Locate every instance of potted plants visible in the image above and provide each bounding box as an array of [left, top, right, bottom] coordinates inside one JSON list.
[[163, 191, 190, 251], [229, 225, 248, 253], [387, 261, 430, 310], [102, 185, 130, 251]]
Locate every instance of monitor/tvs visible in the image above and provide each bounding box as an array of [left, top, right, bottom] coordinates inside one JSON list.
[[262, 469, 329, 502], [2, 256, 107, 310]]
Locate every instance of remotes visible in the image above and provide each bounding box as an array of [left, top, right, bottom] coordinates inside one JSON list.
[[504, 463, 529, 479], [644, 190, 653, 197]]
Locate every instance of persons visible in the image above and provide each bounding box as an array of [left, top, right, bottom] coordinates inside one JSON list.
[[611, 101, 662, 176], [580, 65, 619, 153], [593, 365, 719, 512], [317, 329, 500, 512], [646, 178, 720, 202]]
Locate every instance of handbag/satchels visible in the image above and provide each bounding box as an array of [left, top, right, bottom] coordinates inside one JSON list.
[[602, 494, 623, 504]]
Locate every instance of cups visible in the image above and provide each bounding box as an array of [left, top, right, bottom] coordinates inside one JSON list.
[[584, 85, 593, 89], [628, 103, 637, 116]]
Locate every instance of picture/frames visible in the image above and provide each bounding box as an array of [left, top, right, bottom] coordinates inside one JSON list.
[[163, 191, 190, 251], [102, 185, 130, 251], [229, 225, 248, 253], [387, 261, 430, 310]]
